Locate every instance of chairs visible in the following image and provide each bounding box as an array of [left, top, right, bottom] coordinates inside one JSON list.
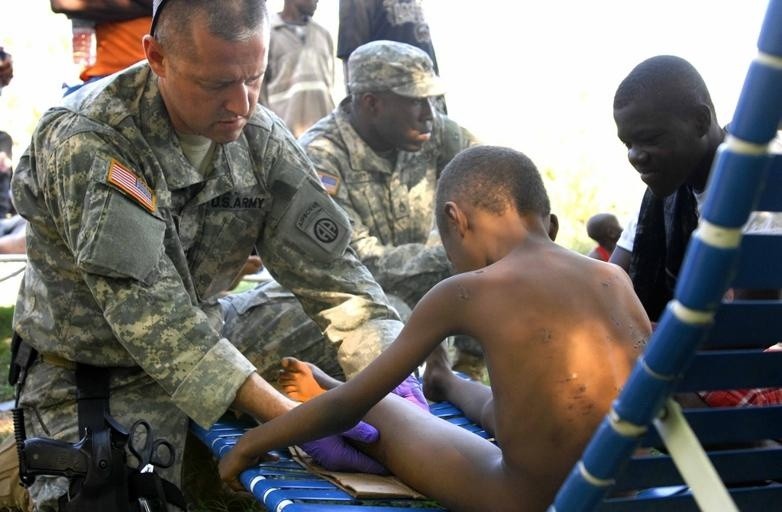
[[190, 0, 782, 512]]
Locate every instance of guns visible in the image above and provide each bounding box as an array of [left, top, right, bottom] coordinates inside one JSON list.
[[23, 427, 126, 511]]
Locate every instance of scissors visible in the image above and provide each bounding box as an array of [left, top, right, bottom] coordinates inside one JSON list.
[[128, 418, 176, 473]]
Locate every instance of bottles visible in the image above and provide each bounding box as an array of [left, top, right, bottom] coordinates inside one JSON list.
[[71, 18, 98, 67]]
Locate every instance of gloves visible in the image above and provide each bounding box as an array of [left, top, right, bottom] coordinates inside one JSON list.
[[393, 376, 428, 410], [298, 421, 388, 474]]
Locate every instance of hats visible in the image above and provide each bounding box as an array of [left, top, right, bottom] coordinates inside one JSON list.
[[347, 39, 446, 98]]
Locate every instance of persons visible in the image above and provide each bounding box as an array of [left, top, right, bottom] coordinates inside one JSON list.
[[587, 55, 782, 489], [0, 0, 480, 321], [13, 0, 427, 511], [216, 145, 651, 511]]
